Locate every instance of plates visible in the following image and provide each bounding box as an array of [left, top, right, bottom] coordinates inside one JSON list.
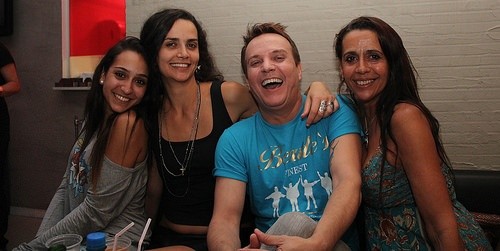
[[44, 233, 83, 251]]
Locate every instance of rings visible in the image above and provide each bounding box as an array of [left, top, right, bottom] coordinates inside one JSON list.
[[319, 100, 326, 114], [326, 98, 334, 109]]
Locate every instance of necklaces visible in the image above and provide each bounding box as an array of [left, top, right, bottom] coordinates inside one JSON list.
[[158, 81, 202, 176]]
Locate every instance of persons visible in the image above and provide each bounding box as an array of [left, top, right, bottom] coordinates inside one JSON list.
[[0, 42, 22, 251], [11, 35, 148, 251], [333, 17, 494, 251], [207, 22, 366, 251], [140, 9, 340, 251]]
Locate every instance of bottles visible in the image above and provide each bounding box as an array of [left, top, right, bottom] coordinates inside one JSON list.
[[48, 244, 66, 251], [86, 232, 107, 251]]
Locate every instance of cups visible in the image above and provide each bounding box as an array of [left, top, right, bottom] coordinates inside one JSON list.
[[105, 237, 133, 251]]
